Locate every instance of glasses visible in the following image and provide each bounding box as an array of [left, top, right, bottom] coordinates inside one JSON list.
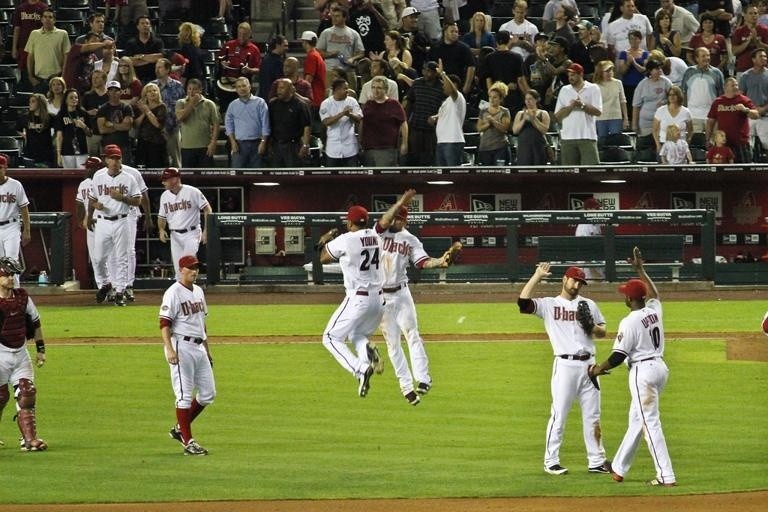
[[118, 64, 129, 67]]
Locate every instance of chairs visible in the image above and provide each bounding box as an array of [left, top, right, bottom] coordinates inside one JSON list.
[[0, 0, 768, 169]]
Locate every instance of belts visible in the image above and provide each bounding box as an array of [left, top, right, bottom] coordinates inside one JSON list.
[[356, 290, 382, 296], [0, 218, 16, 226], [382, 283, 407, 293], [98, 214, 127, 221], [628, 357, 654, 364], [183, 335, 204, 344], [561, 354, 592, 361]]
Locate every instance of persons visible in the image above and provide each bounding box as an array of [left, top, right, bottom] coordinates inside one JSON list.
[[371, 188, 462, 407], [587, 245, 676, 489], [316, 204, 386, 398], [156, 167, 214, 284], [0, 260, 48, 453], [0, 154, 33, 290], [157, 254, 217, 457], [72, 144, 154, 309], [516, 263, 611, 477]]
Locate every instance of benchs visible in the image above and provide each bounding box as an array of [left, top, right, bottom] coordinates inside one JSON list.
[[419, 237, 451, 281], [533, 235, 687, 279], [304, 263, 343, 285]]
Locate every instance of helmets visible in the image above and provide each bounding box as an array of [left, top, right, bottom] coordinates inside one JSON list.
[[158, 167, 180, 182], [0, 256, 24, 277], [80, 156, 102, 169]]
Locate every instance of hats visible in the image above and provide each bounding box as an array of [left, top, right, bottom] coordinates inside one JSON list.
[[347, 205, 368, 223], [617, 276, 647, 302], [533, 19, 593, 73], [401, 6, 421, 19], [178, 255, 202, 269], [106, 80, 122, 90], [564, 265, 588, 286], [584, 199, 600, 210], [103, 144, 122, 158], [393, 203, 408, 222], [0, 155, 9, 168], [298, 30, 318, 41], [496, 30, 510, 44]]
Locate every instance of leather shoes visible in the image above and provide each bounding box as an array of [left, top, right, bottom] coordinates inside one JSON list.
[[170, 227, 196, 233]]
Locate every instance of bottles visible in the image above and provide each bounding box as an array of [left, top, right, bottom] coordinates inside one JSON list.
[[39, 270, 49, 286]]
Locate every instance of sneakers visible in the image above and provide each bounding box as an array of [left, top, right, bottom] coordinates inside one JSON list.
[[646, 478, 676, 487], [183, 439, 209, 456], [416, 382, 430, 393], [612, 472, 624, 482], [96, 282, 136, 306], [405, 390, 420, 405], [358, 362, 373, 398], [588, 461, 611, 474], [168, 426, 187, 448], [366, 341, 384, 375], [543, 464, 569, 476]]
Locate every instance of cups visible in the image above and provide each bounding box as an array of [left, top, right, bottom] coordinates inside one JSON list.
[[161, 268, 168, 278]]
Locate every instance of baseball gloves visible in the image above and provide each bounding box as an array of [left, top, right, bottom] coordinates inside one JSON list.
[[575, 300, 594, 335], [441, 241, 462, 267], [316, 227, 337, 250], [587, 364, 611, 390]]
